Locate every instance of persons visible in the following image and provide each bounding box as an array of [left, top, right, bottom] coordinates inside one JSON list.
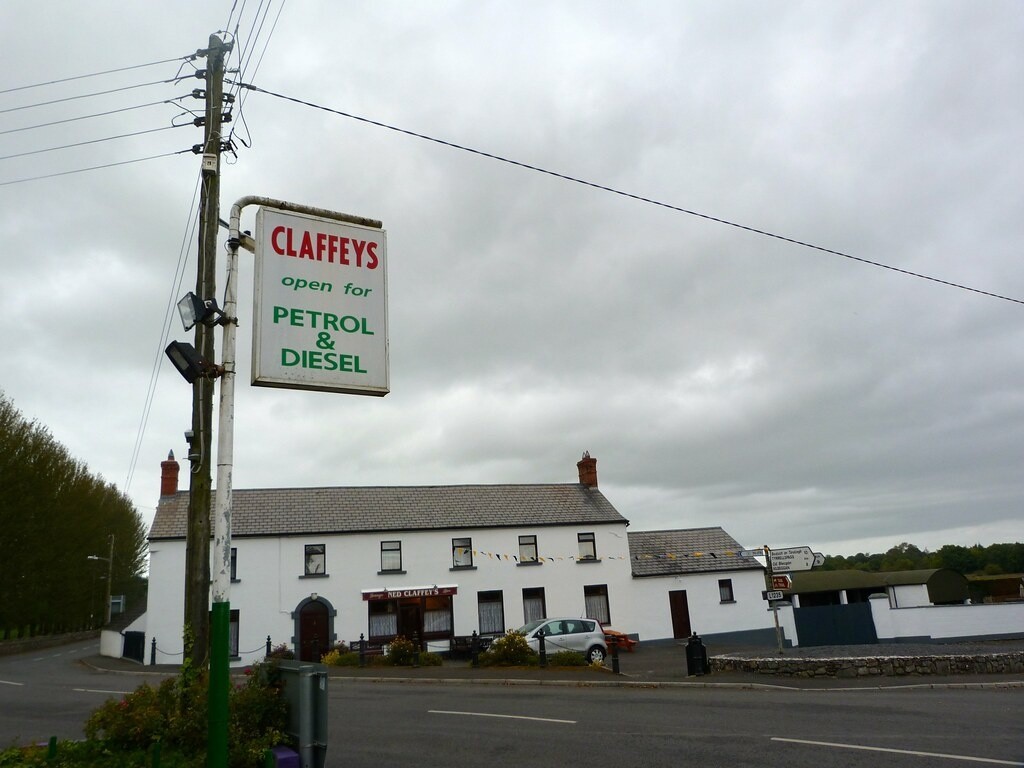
[[246, 666, 291, 751]]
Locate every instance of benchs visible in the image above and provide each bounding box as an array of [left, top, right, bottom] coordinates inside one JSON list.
[[450, 636, 492, 663], [350, 642, 384, 656]]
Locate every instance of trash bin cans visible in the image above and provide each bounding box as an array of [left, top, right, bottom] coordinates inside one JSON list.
[[685, 630, 708, 677]]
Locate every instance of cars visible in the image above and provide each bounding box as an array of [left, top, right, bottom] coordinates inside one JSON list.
[[485, 617, 608, 665]]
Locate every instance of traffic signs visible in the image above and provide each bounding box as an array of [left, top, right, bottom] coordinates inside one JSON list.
[[771, 574, 792, 592], [737, 549, 765, 558], [810, 552, 825, 568], [761, 589, 796, 602], [770, 546, 817, 573]]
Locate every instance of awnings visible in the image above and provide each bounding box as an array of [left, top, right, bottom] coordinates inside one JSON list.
[[362, 584, 459, 601]]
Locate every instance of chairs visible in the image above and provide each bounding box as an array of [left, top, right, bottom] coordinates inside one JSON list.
[[559, 623, 568, 633], [572, 622, 582, 633], [543, 625, 552, 635]]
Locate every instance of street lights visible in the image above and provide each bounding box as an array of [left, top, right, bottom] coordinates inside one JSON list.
[[87, 531, 115, 625]]
